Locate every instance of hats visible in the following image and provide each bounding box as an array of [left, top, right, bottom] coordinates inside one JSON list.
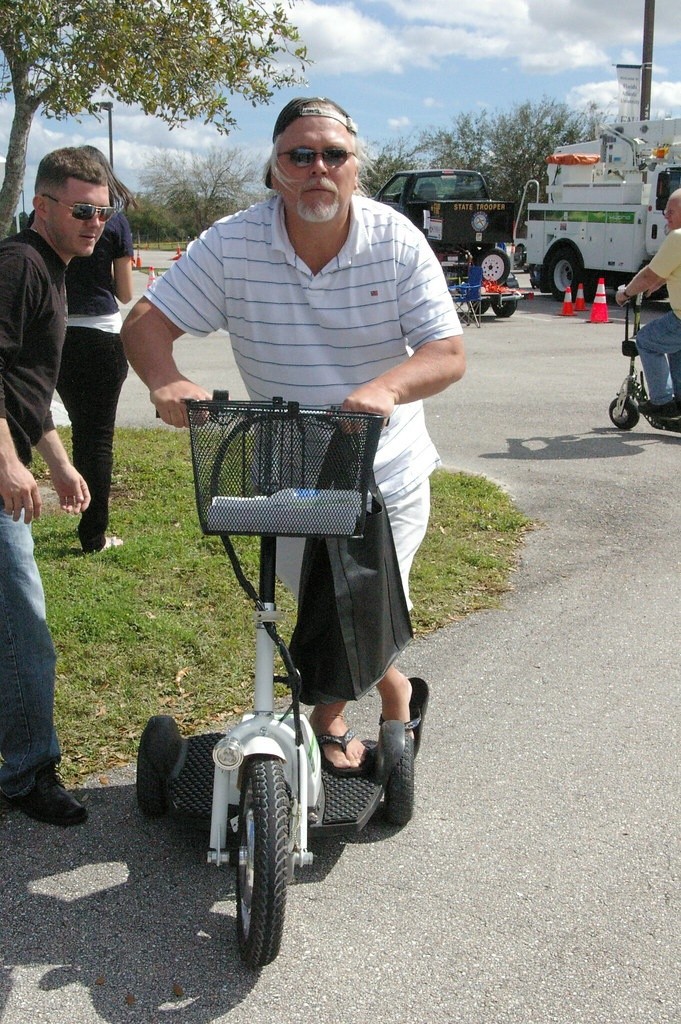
[[265, 96, 357, 190]]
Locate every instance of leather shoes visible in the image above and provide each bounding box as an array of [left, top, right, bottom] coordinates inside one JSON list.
[[0, 765, 88, 826]]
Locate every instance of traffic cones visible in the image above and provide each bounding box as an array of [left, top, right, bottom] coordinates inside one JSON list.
[[556, 284, 578, 316], [572, 282, 589, 311], [171, 254, 182, 260], [584, 278, 613, 324], [175, 243, 182, 254], [146, 266, 156, 290]]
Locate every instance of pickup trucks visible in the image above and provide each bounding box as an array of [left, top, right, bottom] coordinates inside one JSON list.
[[369, 168, 515, 266]]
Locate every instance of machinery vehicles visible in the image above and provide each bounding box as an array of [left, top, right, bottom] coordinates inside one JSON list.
[[510, 116, 680, 305]]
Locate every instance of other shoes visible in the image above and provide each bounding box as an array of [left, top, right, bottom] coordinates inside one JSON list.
[[638, 397, 681, 417], [99, 536, 123, 553]]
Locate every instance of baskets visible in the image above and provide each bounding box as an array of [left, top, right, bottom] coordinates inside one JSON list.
[[186, 400, 386, 539]]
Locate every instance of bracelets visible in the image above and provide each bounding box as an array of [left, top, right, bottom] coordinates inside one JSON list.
[[624, 290, 629, 298]]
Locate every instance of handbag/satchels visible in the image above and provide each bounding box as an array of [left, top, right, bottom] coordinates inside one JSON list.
[[286, 423, 414, 705]]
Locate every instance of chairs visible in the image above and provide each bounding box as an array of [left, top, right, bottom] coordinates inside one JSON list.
[[450, 178, 479, 201], [447, 266, 483, 329], [415, 182, 438, 198]]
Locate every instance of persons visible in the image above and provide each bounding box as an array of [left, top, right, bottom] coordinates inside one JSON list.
[[120, 98, 468, 776], [0, 144, 111, 827], [616, 190, 681, 418], [27, 145, 132, 552]]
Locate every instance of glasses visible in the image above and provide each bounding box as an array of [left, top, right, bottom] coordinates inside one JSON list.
[[276, 147, 359, 167], [42, 193, 116, 222]]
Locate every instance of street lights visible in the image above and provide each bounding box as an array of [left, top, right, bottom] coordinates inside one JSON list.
[[99, 102, 113, 169]]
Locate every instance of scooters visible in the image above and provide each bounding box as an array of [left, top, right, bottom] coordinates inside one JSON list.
[[609, 285, 681, 434], [134, 388, 417, 968]]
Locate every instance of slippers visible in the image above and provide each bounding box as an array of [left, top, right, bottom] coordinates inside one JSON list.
[[316, 727, 375, 777], [378, 677, 429, 759]]
